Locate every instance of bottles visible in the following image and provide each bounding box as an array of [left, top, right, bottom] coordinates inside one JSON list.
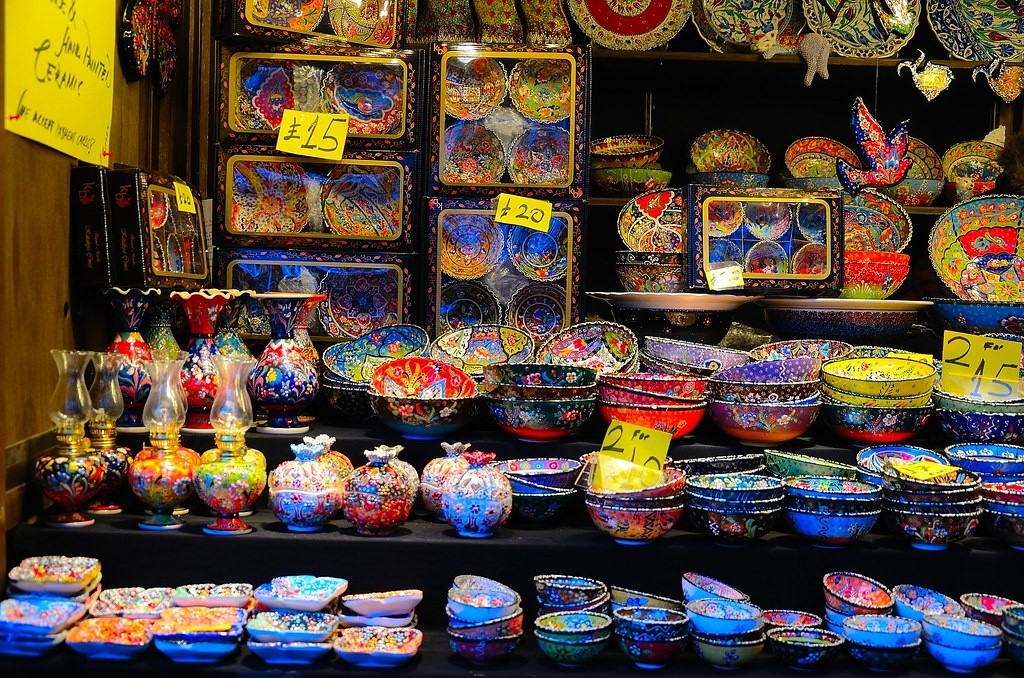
[[271, 435, 514, 540], [107, 281, 320, 434]]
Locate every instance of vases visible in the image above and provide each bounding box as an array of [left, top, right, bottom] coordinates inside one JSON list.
[[99, 286, 325, 435]]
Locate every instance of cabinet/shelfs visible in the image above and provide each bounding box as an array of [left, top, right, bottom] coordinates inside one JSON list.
[[588, 49, 1024, 216]]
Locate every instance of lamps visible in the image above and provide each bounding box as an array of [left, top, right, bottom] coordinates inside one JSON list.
[[29, 349, 267, 534]]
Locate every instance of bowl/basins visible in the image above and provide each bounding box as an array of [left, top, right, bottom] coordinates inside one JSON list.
[[497, 449, 1024, 557], [591, 127, 1016, 297], [856, 444, 947, 467], [945, 443, 1024, 472], [922, 299, 1024, 344], [585, 291, 757, 345], [756, 299, 935, 347], [444, 570, 1024, 674], [321, 321, 1024, 447]]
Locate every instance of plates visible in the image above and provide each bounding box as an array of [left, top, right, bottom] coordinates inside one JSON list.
[[439, 283, 500, 336], [567, 1, 693, 52], [927, 193, 1024, 303], [147, 193, 206, 273], [506, 282, 563, 342], [317, 267, 394, 335], [690, 0, 1024, 67], [234, 56, 572, 282], [1, 556, 424, 668]]
